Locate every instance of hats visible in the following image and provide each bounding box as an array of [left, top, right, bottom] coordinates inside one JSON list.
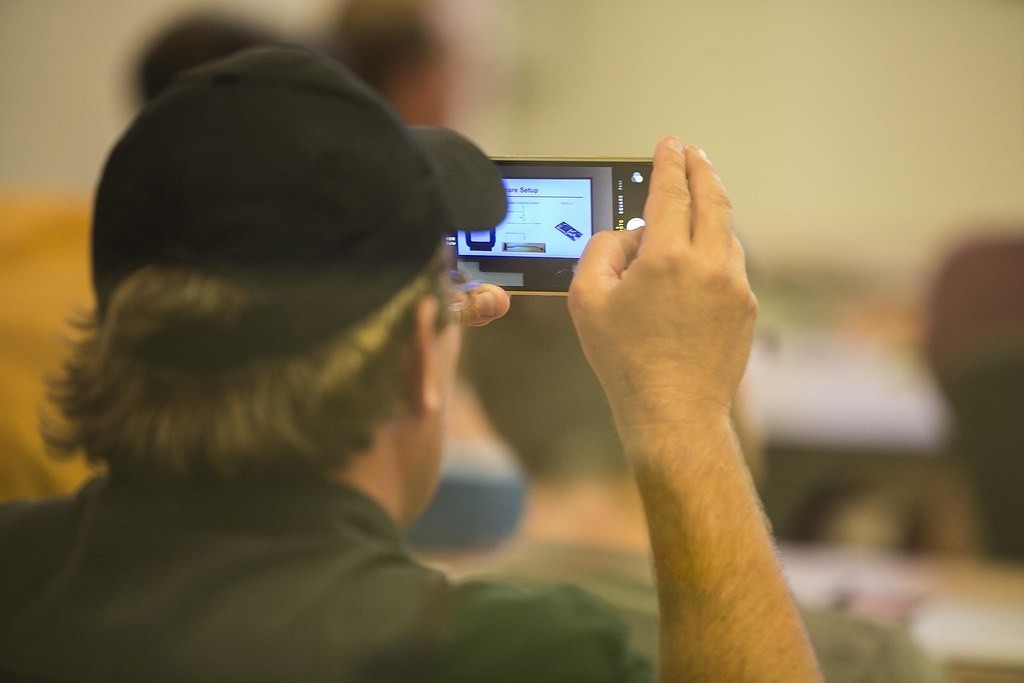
[[89, 45, 509, 366]]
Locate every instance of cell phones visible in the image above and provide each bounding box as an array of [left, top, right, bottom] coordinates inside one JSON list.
[[445, 157, 654, 296]]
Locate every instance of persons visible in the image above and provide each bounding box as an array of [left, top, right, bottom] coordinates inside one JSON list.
[[407, 207, 930, 683], [821, 236, 1024, 564], [0, 0, 455, 499], [0, 45, 826, 683]]
